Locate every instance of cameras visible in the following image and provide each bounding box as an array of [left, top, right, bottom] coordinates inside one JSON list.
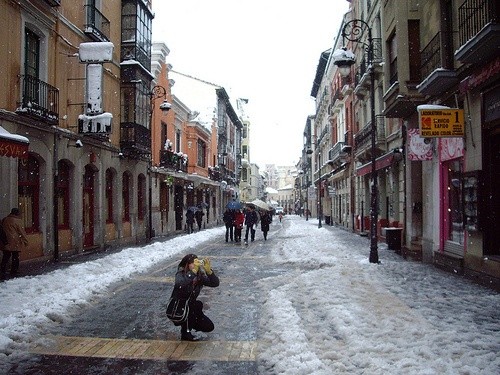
[[198, 259, 204, 267]]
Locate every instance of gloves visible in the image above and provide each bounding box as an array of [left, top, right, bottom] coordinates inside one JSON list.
[[192, 258, 200, 274], [200, 257, 213, 275]]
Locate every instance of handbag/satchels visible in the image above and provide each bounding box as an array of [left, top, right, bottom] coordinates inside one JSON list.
[[166, 301, 189, 322], [252, 224, 257, 230]]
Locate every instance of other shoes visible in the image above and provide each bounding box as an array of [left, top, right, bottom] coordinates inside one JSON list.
[[244, 241, 247, 244], [181, 333, 199, 340]]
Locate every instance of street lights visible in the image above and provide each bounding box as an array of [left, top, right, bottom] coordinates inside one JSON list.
[[305, 143, 322, 229], [333, 18, 383, 264], [147, 85, 172, 241]]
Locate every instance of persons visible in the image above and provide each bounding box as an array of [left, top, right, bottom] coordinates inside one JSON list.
[[223, 205, 271, 244], [0, 208, 29, 280], [186, 210, 195, 234], [170, 254, 219, 341], [279, 213, 283, 222], [195, 207, 205, 230]]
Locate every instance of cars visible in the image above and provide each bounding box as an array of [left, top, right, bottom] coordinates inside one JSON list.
[[274, 206, 285, 216]]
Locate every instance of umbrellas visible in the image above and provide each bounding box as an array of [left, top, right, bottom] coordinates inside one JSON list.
[[188, 206, 198, 214], [225, 199, 243, 209], [252, 199, 269, 210], [196, 201, 208, 207]]
